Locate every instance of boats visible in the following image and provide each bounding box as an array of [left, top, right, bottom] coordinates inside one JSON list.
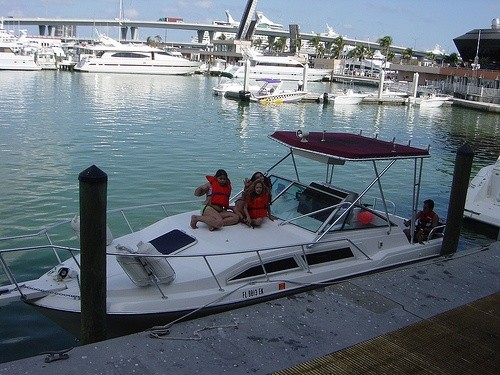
[[0, 23, 500, 112], [0, 121, 447, 344], [463, 155, 500, 228]]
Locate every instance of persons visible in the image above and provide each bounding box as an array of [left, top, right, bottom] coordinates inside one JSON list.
[[233, 171, 278, 230], [297, 79, 303, 91], [190, 168, 240, 231], [401, 199, 440, 244]]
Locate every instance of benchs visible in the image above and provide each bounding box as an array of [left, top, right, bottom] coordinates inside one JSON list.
[[297, 181, 357, 225]]
